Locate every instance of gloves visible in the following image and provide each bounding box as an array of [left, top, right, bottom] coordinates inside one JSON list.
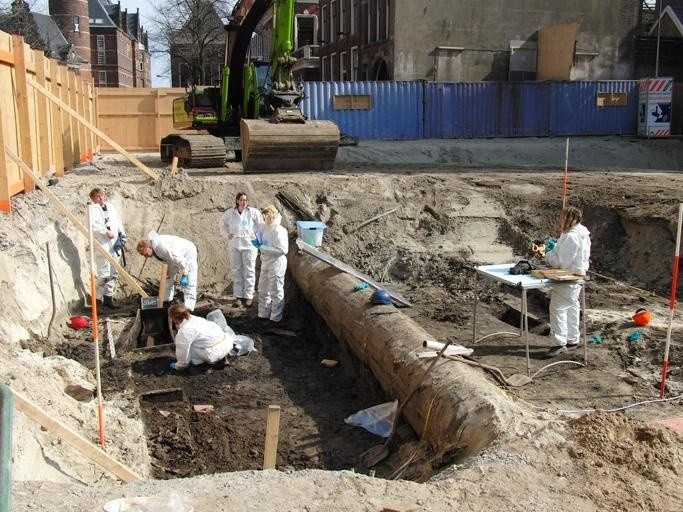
[[591, 336, 603, 343], [354, 282, 368, 291], [546, 240, 554, 252], [180, 275, 188, 285], [629, 332, 641, 341], [252, 239, 260, 246], [170, 363, 176, 369]]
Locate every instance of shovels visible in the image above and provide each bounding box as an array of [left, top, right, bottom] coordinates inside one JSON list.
[[360, 337, 453, 468], [436, 352, 533, 386]]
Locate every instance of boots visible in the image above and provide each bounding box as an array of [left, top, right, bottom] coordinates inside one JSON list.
[[103, 295, 120, 309], [96, 299, 105, 314]]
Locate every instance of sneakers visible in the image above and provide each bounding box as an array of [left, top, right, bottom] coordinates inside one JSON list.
[[233, 298, 253, 307], [215, 357, 229, 369], [544, 342, 577, 357]]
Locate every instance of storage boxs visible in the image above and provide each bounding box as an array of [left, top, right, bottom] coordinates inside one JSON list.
[[295, 220, 327, 247]]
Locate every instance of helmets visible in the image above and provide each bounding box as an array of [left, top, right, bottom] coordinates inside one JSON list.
[[70, 316, 89, 329], [632, 310, 650, 326], [369, 290, 390, 304]]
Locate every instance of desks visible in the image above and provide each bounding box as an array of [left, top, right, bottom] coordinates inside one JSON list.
[[473, 262, 587, 377]]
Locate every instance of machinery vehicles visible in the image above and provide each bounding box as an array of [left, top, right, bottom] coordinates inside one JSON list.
[[156, 0, 342, 174]]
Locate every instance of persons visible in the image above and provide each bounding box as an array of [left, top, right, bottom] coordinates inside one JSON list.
[[250, 205, 289, 329], [88, 187, 127, 311], [218, 190, 263, 306], [135, 230, 198, 312], [543, 204, 592, 358], [166, 304, 233, 372]]
